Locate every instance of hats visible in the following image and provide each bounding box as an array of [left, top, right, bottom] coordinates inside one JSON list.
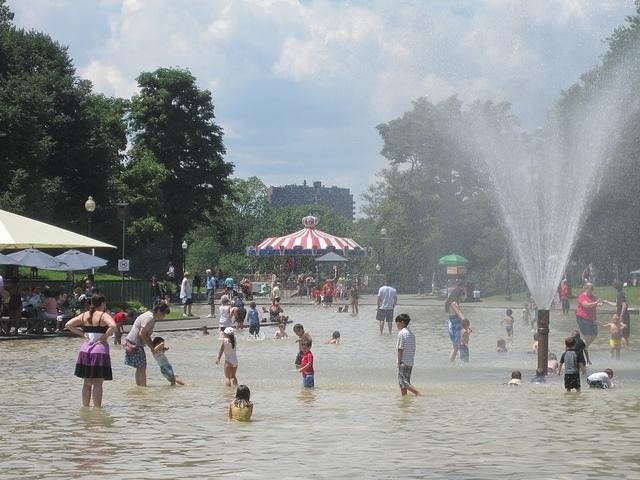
[[221, 294, 228, 302], [224, 327, 234, 335]]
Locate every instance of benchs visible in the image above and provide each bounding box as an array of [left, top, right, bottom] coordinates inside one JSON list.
[[0, 308, 75, 336]]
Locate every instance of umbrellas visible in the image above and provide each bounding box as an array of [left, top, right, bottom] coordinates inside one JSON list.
[[437, 252, 470, 265], [5, 248, 54, 278], [40, 248, 109, 286], [0, 252, 22, 265], [0, 206, 117, 250]]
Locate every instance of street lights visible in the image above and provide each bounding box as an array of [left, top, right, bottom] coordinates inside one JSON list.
[[503, 214, 514, 301], [380, 224, 387, 273], [84, 195, 96, 279], [375, 263, 380, 294], [181, 240, 188, 274]]
